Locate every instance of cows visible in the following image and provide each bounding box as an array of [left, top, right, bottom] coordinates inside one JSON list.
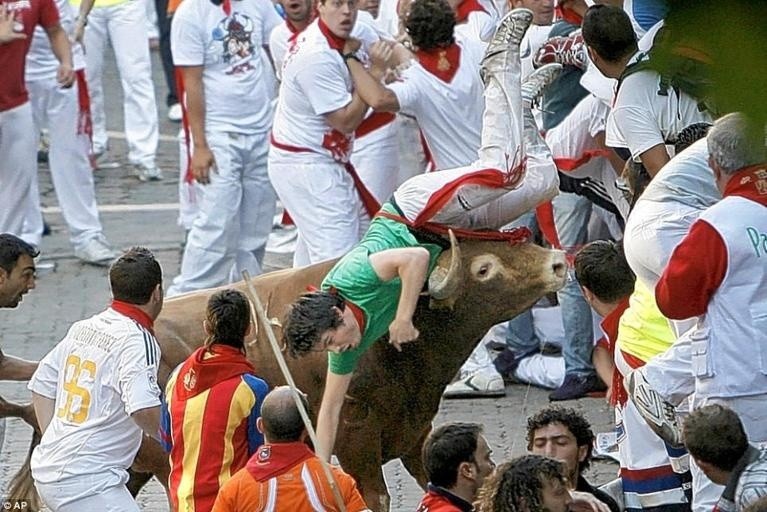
[[0, 228, 568, 512]]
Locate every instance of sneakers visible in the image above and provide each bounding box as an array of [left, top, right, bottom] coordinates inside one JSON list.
[[442, 369, 506, 397], [549, 373, 597, 401], [169, 103, 184, 120], [73, 237, 115, 263], [485, 7, 585, 106], [493, 346, 541, 374], [135, 162, 164, 182]]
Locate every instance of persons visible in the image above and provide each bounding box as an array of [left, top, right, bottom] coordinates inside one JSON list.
[[0, 1, 765, 511]]
